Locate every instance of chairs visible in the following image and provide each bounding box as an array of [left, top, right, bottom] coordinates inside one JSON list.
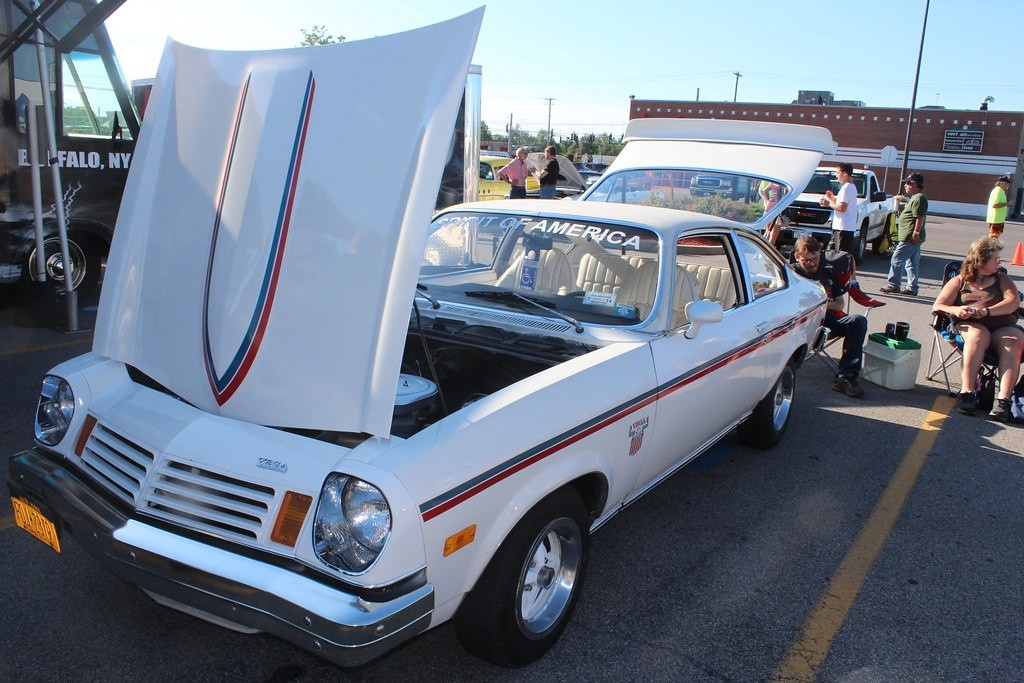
[[789, 248, 886, 376], [497, 247, 573, 296], [618, 261, 700, 327], [926, 261, 1024, 399]]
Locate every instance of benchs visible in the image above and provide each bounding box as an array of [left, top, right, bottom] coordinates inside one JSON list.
[[573, 253, 737, 324]]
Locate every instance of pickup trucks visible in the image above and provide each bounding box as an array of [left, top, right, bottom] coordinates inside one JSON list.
[[757, 167, 899, 266]]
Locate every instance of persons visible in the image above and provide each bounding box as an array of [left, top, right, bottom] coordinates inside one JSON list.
[[529, 145, 559, 199], [789, 236, 867, 397], [497, 147, 528, 199], [820, 163, 857, 253], [759, 181, 788, 245], [880, 172, 928, 296], [933, 236, 1024, 422], [987, 176, 1016, 238]]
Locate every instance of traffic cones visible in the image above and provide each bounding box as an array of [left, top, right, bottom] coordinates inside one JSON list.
[[1009, 240, 1024, 266]]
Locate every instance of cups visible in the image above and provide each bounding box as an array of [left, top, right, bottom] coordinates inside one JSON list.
[[896, 322, 910, 341], [819, 195, 826, 206]]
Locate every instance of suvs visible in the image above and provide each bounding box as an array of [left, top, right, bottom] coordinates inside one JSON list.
[[690, 172, 758, 202]]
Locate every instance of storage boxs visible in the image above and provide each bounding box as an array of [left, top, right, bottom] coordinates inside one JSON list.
[[864, 333, 921, 390]]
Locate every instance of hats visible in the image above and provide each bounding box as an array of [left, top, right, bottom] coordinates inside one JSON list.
[[901, 173, 924, 185], [998, 175, 1012, 183]]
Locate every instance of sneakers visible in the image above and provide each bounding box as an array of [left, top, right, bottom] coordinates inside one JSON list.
[[833, 374, 864, 398], [989, 398, 1013, 422], [957, 390, 976, 414]]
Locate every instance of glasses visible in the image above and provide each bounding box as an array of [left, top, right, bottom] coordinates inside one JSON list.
[[798, 251, 821, 263], [986, 256, 1001, 264]]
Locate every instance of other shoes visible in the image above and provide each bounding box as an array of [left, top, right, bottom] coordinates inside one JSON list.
[[879, 285, 900, 293], [899, 286, 917, 296]]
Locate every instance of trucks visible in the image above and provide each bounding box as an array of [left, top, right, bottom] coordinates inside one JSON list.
[[0, 0, 145, 322]]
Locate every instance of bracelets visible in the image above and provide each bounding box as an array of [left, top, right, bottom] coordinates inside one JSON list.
[[915, 231, 919, 234], [986, 307, 990, 316]]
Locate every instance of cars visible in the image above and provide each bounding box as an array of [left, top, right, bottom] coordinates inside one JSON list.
[[6, 3, 838, 673], [476, 146, 657, 201]]
